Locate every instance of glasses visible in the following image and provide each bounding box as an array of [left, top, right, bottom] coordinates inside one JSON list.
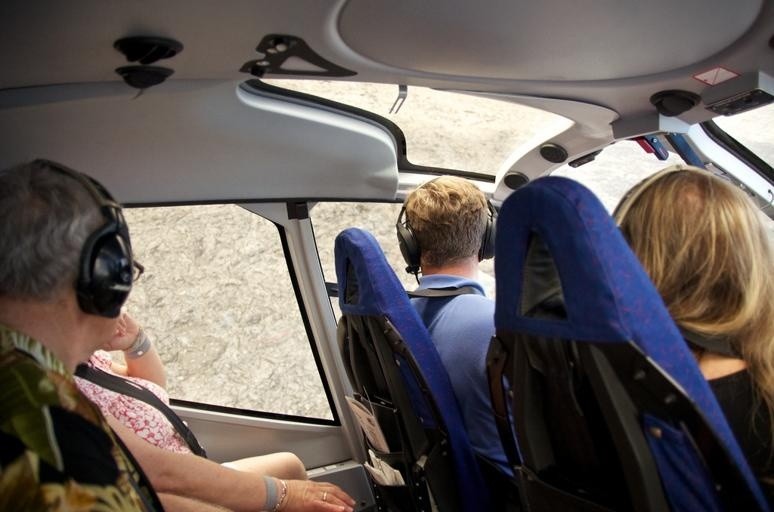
[[133, 260, 144, 283]]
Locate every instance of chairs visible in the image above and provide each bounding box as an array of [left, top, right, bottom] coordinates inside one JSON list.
[[494, 176, 768, 507], [334, 228, 499, 508]]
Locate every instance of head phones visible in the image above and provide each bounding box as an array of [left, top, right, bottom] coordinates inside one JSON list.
[[396, 175, 499, 274], [31, 160, 132, 318]]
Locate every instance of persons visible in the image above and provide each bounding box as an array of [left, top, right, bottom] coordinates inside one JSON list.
[[400, 175, 525, 512], [2, 160, 357, 512], [613, 165, 774, 512]]
